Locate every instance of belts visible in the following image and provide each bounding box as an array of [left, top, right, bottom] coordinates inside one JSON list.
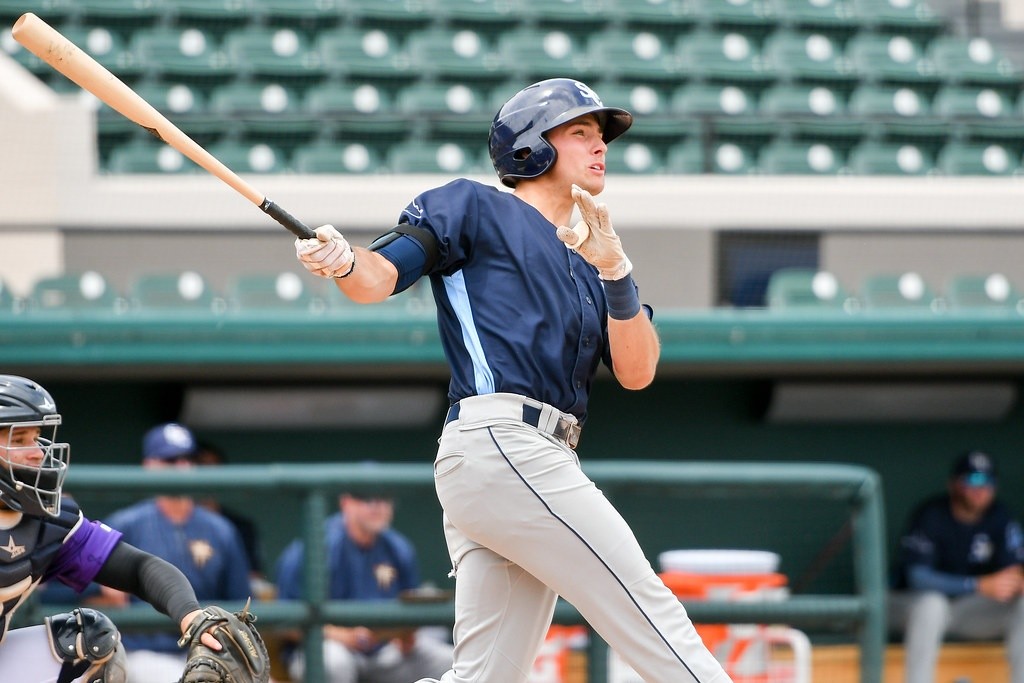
[[445, 400, 581, 450]]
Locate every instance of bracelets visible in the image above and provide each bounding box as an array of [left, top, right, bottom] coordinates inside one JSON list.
[[332, 245, 355, 279]]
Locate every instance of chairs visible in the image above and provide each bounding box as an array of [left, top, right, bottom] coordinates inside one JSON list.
[[123, 270, 210, 315], [861, 275, 940, 306], [26, 268, 113, 314], [232, 269, 314, 312], [955, 272, 1020, 308], [1, 2, 1024, 177], [766, 266, 847, 308]]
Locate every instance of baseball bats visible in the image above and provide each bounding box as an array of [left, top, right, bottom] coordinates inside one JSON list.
[[11, 11, 316, 239]]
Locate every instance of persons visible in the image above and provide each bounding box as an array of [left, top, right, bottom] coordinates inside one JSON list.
[[1, 376, 271, 683], [288, 481, 459, 683], [889, 447, 1024, 682], [294, 78, 736, 683]]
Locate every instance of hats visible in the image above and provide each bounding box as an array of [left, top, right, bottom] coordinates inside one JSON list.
[[951, 447, 995, 482], [141, 424, 195, 459]]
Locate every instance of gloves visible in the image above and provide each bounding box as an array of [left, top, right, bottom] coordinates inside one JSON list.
[[293, 224, 354, 279], [555, 181, 634, 281]]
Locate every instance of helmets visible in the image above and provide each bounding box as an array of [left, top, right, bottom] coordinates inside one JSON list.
[[0, 371, 70, 520], [488, 77, 635, 187]]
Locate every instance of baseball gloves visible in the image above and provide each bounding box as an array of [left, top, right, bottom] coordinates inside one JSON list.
[[173, 603, 276, 683]]
